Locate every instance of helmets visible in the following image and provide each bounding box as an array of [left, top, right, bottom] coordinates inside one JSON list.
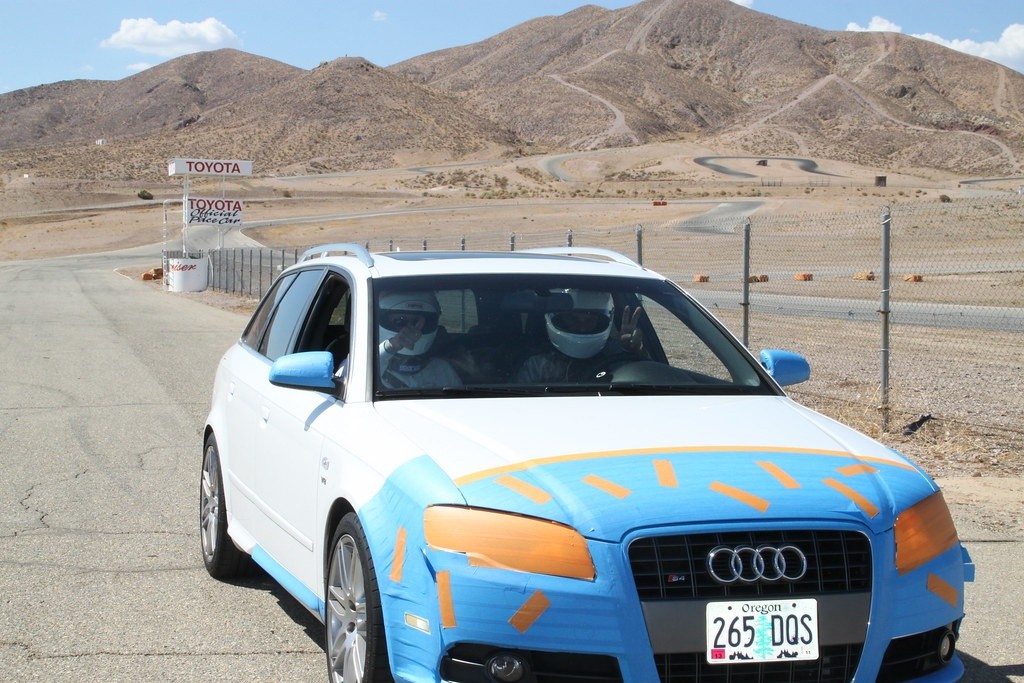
[[544, 289, 615, 359], [379, 290, 441, 374]]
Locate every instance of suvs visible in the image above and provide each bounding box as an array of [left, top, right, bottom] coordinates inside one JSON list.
[[196, 241, 977, 682]]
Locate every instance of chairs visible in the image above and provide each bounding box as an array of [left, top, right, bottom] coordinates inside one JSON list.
[[323, 292, 351, 375]]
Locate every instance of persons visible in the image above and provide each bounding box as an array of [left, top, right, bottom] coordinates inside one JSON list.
[[333, 288, 494, 394], [515, 287, 654, 384]]
